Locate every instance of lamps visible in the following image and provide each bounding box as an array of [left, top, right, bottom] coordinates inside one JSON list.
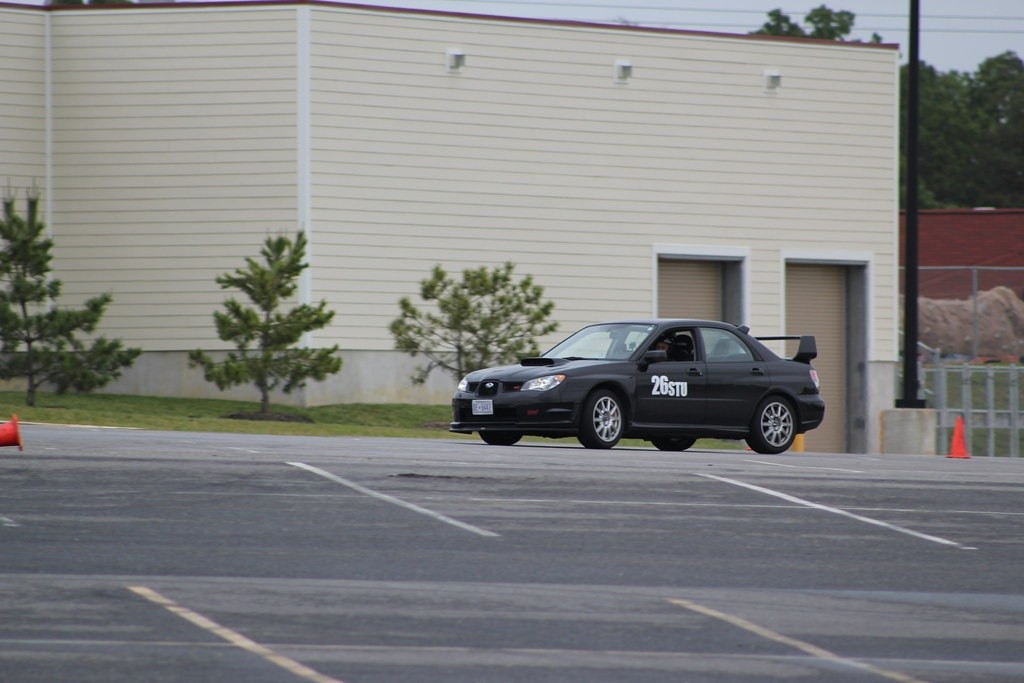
[[447, 49, 465, 71], [763, 71, 786, 93], [611, 62, 632, 84]]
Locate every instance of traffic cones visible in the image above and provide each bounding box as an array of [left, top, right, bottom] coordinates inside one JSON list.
[[947, 414, 972, 459], [792, 431, 804, 453], [0, 412, 24, 454]]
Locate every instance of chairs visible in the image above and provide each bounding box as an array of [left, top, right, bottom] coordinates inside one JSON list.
[[668, 333, 695, 362]]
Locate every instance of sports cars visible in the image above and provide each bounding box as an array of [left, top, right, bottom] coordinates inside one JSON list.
[[450, 319, 825, 453]]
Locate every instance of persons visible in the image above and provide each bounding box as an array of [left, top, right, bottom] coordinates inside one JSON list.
[[896, 350, 928, 408], [655, 334, 675, 351]]
